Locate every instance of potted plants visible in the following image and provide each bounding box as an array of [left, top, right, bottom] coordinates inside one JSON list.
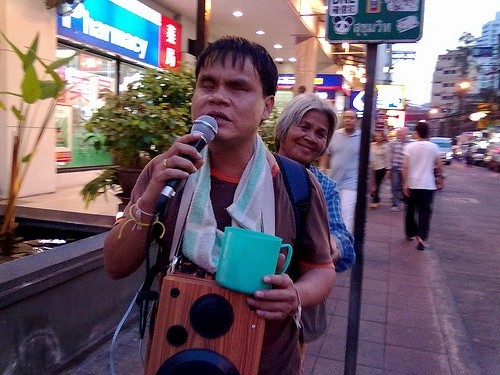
[[78, 61, 200, 210]]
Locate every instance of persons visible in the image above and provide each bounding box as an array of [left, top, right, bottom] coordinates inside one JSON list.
[[318, 107, 360, 242], [101, 36, 338, 375], [266, 94, 361, 281], [401, 122, 444, 250], [367, 125, 413, 212]]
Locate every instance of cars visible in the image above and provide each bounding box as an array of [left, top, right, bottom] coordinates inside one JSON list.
[[453, 144, 487, 162]]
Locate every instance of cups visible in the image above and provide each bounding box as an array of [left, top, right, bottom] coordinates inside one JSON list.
[[215, 227, 294, 295]]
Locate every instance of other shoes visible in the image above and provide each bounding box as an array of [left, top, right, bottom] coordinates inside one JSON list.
[[370, 203, 377, 207], [408, 237, 416, 239], [391, 207, 400, 212], [417, 239, 425, 250]]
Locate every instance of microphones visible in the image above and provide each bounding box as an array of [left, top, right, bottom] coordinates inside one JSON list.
[[158, 114, 218, 204]]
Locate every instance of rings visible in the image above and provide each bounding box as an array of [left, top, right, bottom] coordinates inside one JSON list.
[[162, 158, 168, 167]]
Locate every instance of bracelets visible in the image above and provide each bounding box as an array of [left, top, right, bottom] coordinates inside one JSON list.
[[132, 196, 156, 218], [128, 207, 161, 228]]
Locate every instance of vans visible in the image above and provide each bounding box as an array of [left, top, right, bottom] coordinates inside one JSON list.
[[431, 137, 455, 165]]
